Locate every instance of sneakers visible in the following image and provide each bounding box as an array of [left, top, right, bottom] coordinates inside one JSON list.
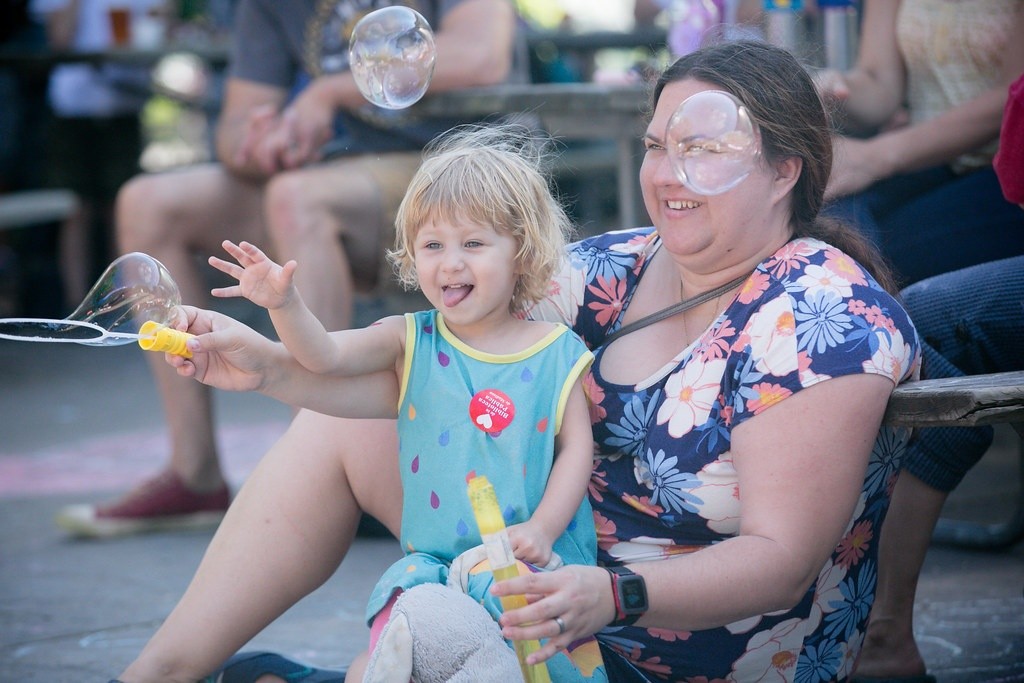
[[55, 468, 228, 540], [197, 651, 346, 683], [356, 512, 394, 538]]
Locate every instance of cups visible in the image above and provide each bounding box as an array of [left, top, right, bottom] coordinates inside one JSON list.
[[109, 9, 130, 47]]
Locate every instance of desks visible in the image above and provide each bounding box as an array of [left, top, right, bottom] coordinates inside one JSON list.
[[420, 80, 659, 232]]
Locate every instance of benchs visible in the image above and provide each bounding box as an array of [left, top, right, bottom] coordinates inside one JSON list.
[[883, 370, 1023, 443]]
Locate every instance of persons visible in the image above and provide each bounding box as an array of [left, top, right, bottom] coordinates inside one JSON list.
[[54, 0, 515, 541], [810, 0, 1024, 291], [33, 0, 169, 322], [107, 40, 918, 683], [851, 77, 1024, 683]]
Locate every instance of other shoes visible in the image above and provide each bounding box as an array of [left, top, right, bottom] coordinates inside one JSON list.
[[847, 672, 937, 683]]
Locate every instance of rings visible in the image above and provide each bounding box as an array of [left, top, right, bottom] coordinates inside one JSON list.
[[554, 617, 565, 634]]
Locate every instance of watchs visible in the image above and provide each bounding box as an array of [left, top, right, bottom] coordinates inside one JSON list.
[[601, 565, 650, 627]]
